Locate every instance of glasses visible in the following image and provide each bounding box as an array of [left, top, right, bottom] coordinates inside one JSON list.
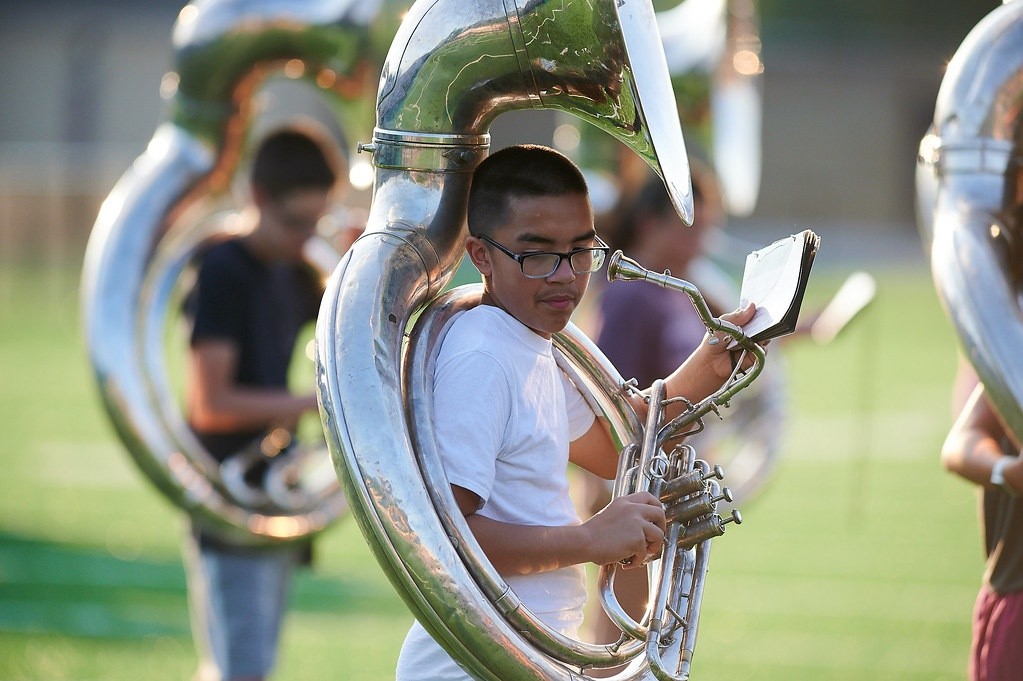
[[477, 231, 611, 279]]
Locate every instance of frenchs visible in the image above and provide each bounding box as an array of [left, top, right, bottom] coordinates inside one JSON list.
[[79, 0, 384, 548], [910, 0, 1023, 456], [310, 0, 765, 680]]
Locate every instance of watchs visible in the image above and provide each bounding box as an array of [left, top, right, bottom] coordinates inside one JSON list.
[[990, 455, 1018, 498]]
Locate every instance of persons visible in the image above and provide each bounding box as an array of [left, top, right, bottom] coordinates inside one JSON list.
[[397, 143, 766, 680], [580, 151, 729, 679], [938, 93, 1023, 681], [179, 125, 364, 681]]
